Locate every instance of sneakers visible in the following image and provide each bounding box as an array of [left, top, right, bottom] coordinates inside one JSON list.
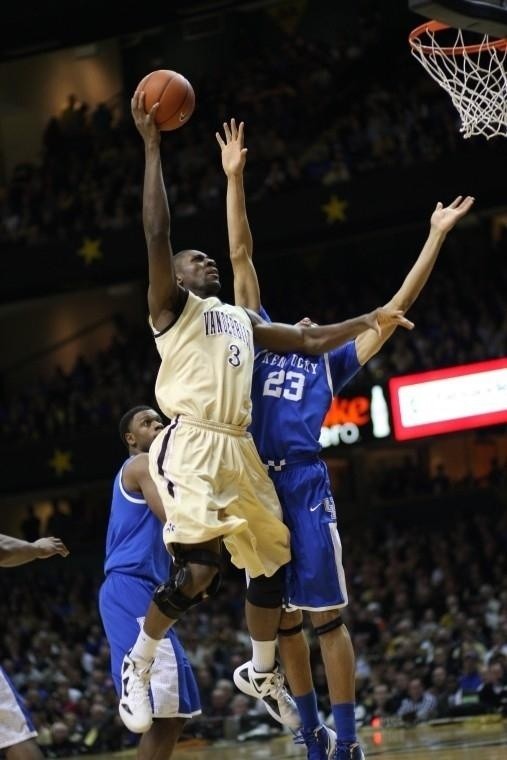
[[118, 647, 157, 734], [332, 740, 366, 760], [233, 658, 302, 729], [292, 722, 338, 760]]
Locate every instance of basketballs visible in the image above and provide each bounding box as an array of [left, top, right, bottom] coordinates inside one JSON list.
[[136, 70, 196, 131]]
